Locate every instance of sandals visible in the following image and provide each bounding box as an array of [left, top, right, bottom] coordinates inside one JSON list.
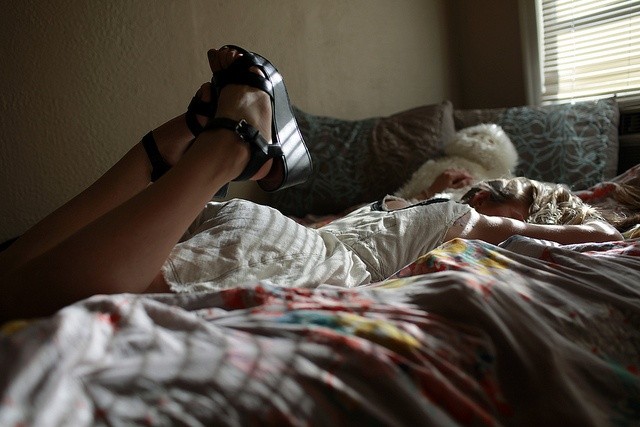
[[142, 79, 216, 183], [203, 43, 314, 193]]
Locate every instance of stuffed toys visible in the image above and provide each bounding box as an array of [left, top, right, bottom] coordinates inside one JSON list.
[[394, 122, 520, 202]]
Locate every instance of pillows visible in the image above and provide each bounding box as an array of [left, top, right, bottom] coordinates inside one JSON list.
[[451, 96, 620, 200], [271, 98, 456, 210]]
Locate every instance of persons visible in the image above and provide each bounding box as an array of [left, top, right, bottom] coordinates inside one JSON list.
[[1, 45, 625, 318]]
